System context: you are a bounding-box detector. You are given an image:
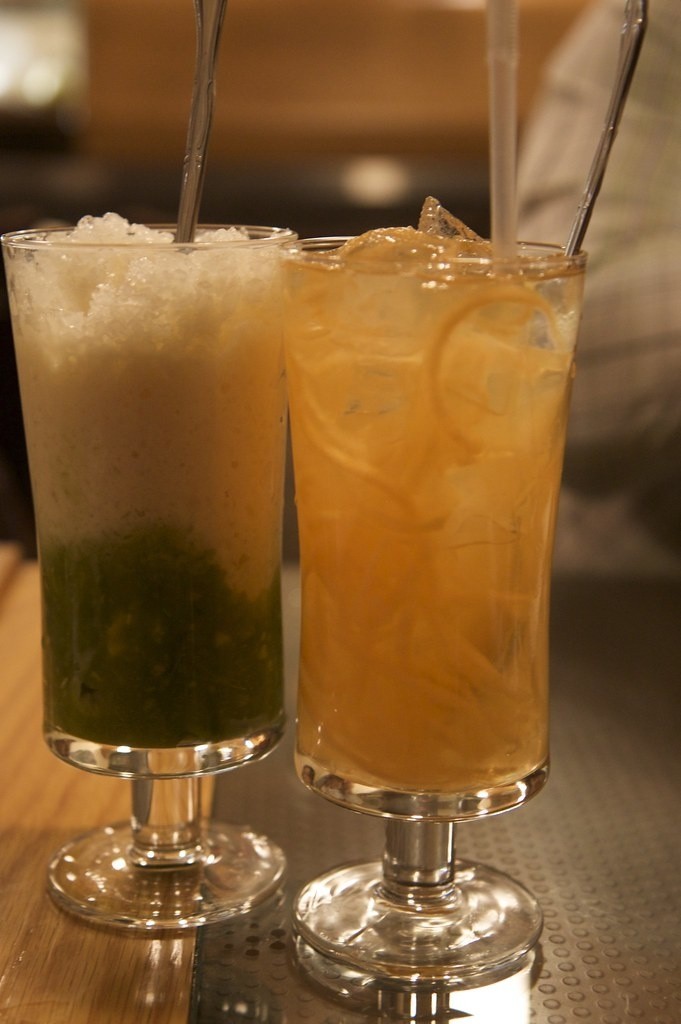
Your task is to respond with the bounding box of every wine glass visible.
[282,235,587,983]
[4,223,297,930]
